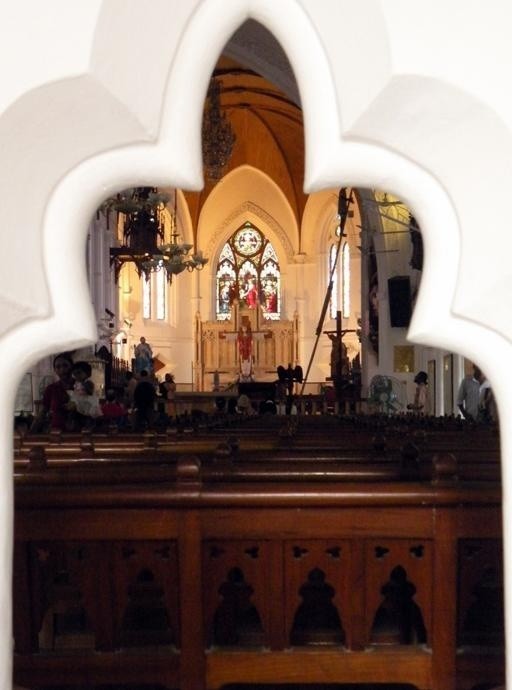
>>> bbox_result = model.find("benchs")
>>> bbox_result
[14,415,503,689]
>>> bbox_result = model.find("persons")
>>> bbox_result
[407,372,428,417]
[134,337,153,373]
[456,364,491,433]
[14,354,176,435]
[325,329,348,377]
[223,323,269,377]
[338,188,349,237]
[230,394,277,417]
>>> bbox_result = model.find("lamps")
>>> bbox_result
[99,187,208,284]
[203,71,237,181]
